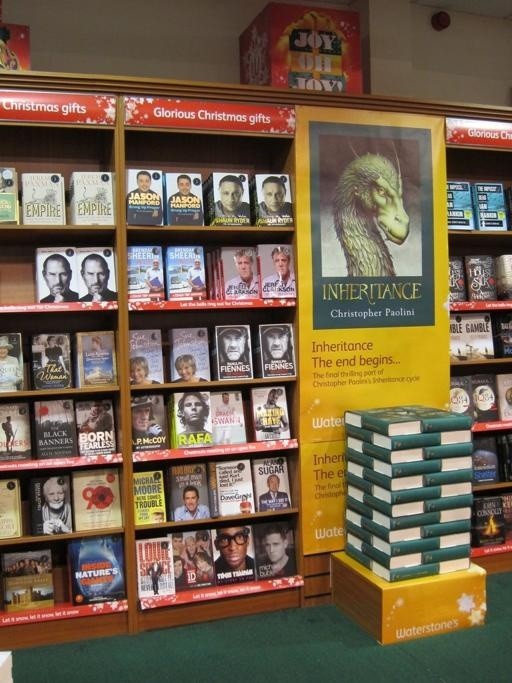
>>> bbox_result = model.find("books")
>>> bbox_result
[126,170,297,302]
[128,323,296,452]
[447,180,512,548]
[132,457,297,598]
[0,468,127,615]
[0,330,118,462]
[0,168,118,304]
[344,404,474,583]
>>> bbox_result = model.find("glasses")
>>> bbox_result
[215,532,248,548]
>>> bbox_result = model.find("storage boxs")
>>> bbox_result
[240,1,364,94]
